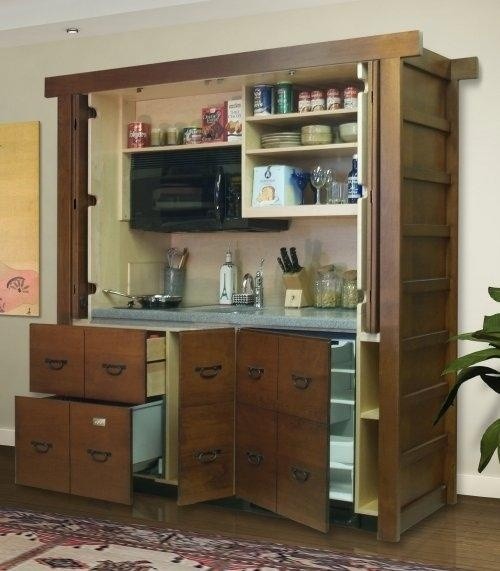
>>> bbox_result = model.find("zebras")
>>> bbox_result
[130,148,290,233]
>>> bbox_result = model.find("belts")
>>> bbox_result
[292,165,335,205]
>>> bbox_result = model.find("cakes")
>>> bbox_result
[183,126,202,145]
[298,91,311,112]
[274,81,295,114]
[127,121,151,148]
[326,88,341,110]
[344,86,358,109]
[311,91,325,111]
[252,84,271,116]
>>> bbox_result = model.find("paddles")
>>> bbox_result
[1,507,463,571]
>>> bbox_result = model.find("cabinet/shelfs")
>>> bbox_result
[11,319,236,521]
[347,155,363,204]
[311,265,363,310]
[163,268,186,296]
[240,82,359,221]
[218,247,237,305]
[239,321,356,534]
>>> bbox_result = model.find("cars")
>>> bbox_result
[336,183,348,203]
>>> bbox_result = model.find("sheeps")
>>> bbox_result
[241,270,264,307]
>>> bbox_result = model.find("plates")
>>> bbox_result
[240,82,359,221]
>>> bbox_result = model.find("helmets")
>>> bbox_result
[261,132,301,149]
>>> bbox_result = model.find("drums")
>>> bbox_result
[275,245,301,274]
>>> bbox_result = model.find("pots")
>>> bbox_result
[102,289,183,310]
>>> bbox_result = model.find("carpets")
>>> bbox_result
[1,507,463,571]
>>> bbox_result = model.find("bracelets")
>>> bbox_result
[300,122,358,144]
